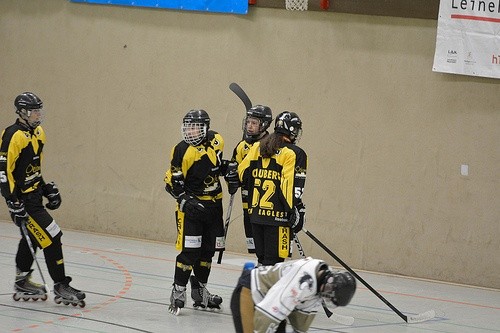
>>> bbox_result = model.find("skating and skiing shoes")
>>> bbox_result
[168,283,186,317]
[13,267,48,302]
[52,276,87,307]
[191,285,224,312]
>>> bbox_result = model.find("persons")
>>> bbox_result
[238,111,308,265]
[0,92,86,308]
[230,258,356,333]
[225,104,274,254]
[163,110,238,317]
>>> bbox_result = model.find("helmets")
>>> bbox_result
[180,109,211,147]
[13,92,46,129]
[241,104,275,139]
[274,111,304,145]
[317,270,357,310]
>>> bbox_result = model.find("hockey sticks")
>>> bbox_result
[216,81,253,265]
[301,226,436,324]
[292,232,355,327]
[21,221,51,302]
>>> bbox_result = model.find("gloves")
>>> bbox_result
[289,203,306,236]
[6,199,29,226]
[41,181,62,211]
[177,195,206,221]
[223,160,240,195]
